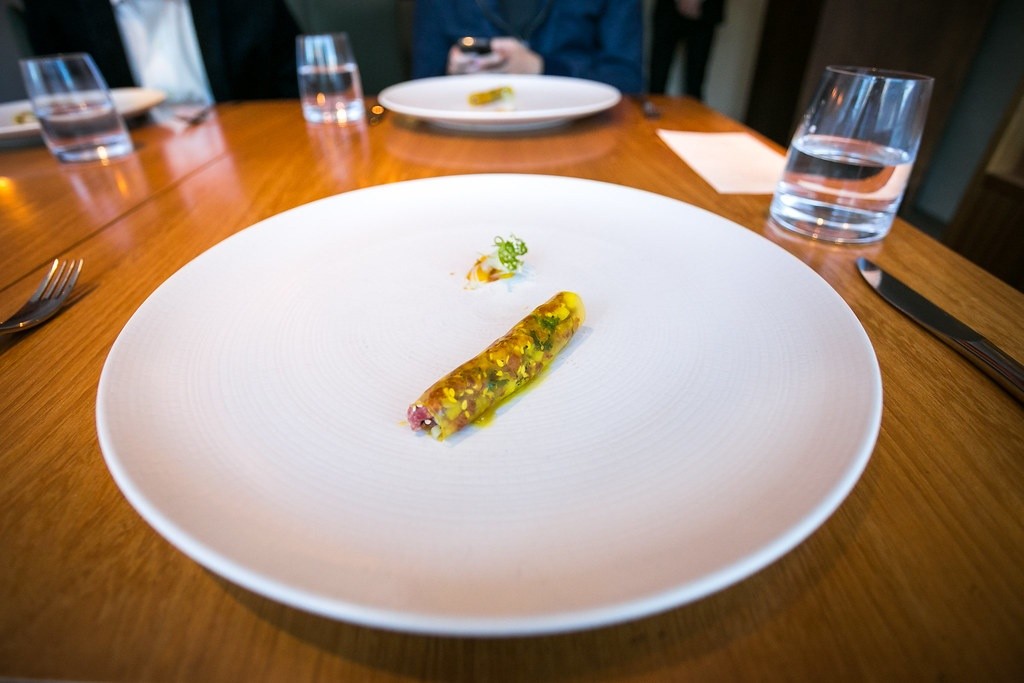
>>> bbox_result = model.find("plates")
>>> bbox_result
[378,74,621,132]
[96,175,882,635]
[0,88,168,150]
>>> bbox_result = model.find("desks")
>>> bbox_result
[0,99,291,290]
[0,94,1024,683]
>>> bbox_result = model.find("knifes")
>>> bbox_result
[856,258,1024,401]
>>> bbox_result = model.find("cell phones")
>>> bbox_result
[459,37,491,55]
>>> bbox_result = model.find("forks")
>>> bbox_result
[0,258,85,336]
[178,107,213,126]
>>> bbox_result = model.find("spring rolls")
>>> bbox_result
[467,87,512,105]
[406,291,586,442]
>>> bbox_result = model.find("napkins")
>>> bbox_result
[655,128,786,195]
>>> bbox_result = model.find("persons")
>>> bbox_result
[649,0,726,104]
[411,0,643,94]
[22,0,306,107]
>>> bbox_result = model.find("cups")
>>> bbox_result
[295,31,365,128]
[20,54,133,168]
[769,66,934,244]
